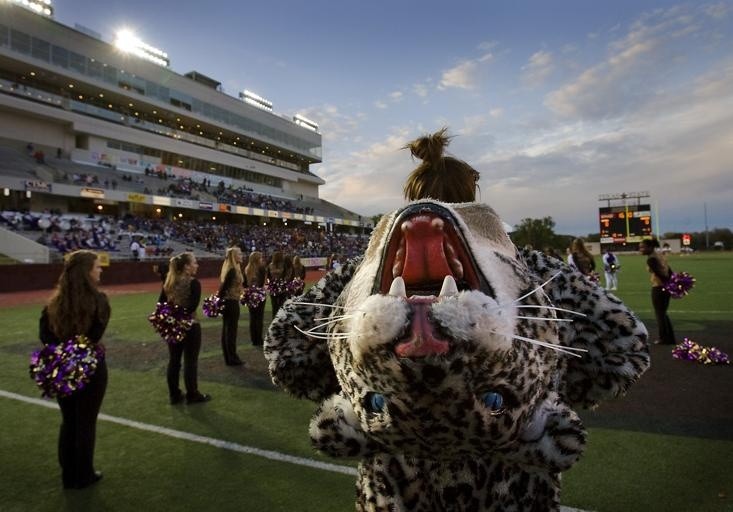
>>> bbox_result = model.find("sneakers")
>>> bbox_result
[200,392,211,401]
[94,470,102,480]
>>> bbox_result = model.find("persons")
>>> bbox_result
[267,249,289,320]
[243,249,267,347]
[36,247,114,491]
[292,255,306,298]
[1,141,371,271]
[541,244,565,264]
[152,247,211,407]
[215,245,245,366]
[601,245,621,292]
[637,236,677,347]
[568,237,597,283]
[283,254,294,299]
[259,124,655,511]
